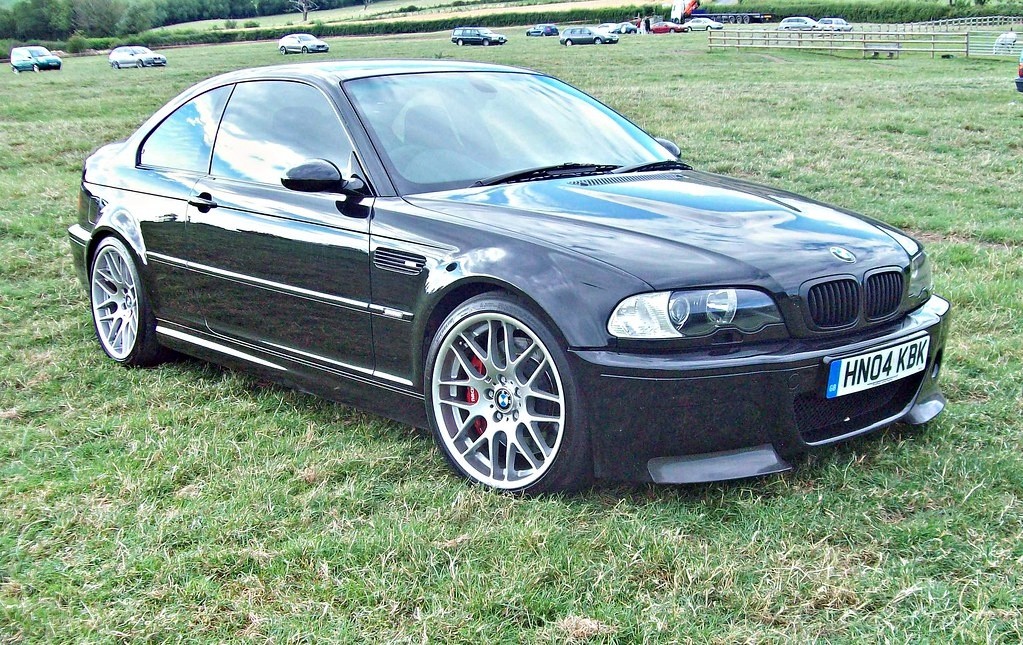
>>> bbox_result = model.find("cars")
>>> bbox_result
[109,47,167,69]
[816,18,854,32]
[277,34,327,55]
[560,27,620,47]
[597,22,638,34]
[450,26,508,46]
[652,20,687,31]
[9,47,61,71]
[780,17,820,31]
[685,17,723,30]
[525,24,561,36]
[63,59,953,497]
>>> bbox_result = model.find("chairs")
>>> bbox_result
[404,104,463,156]
[268,106,350,167]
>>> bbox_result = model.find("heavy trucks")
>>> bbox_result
[671,1,774,23]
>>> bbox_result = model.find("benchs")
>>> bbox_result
[863,45,902,59]
